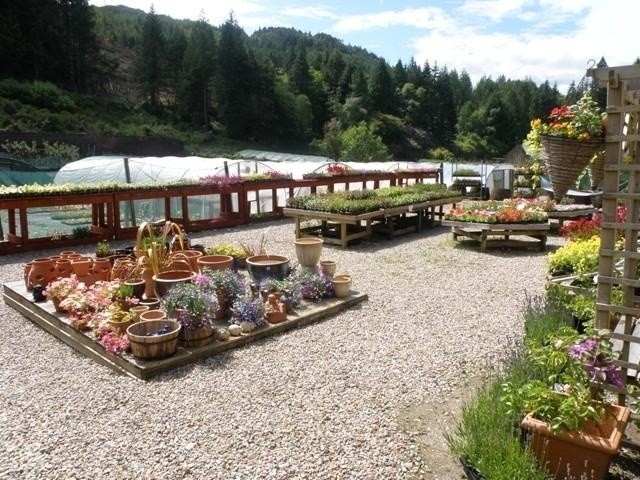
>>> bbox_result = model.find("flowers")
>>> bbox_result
[522,96,608,162]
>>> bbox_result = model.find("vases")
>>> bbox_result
[21,218,356,362]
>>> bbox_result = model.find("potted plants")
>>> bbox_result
[445,198,638,480]
[511,164,546,199]
[1,160,483,245]
